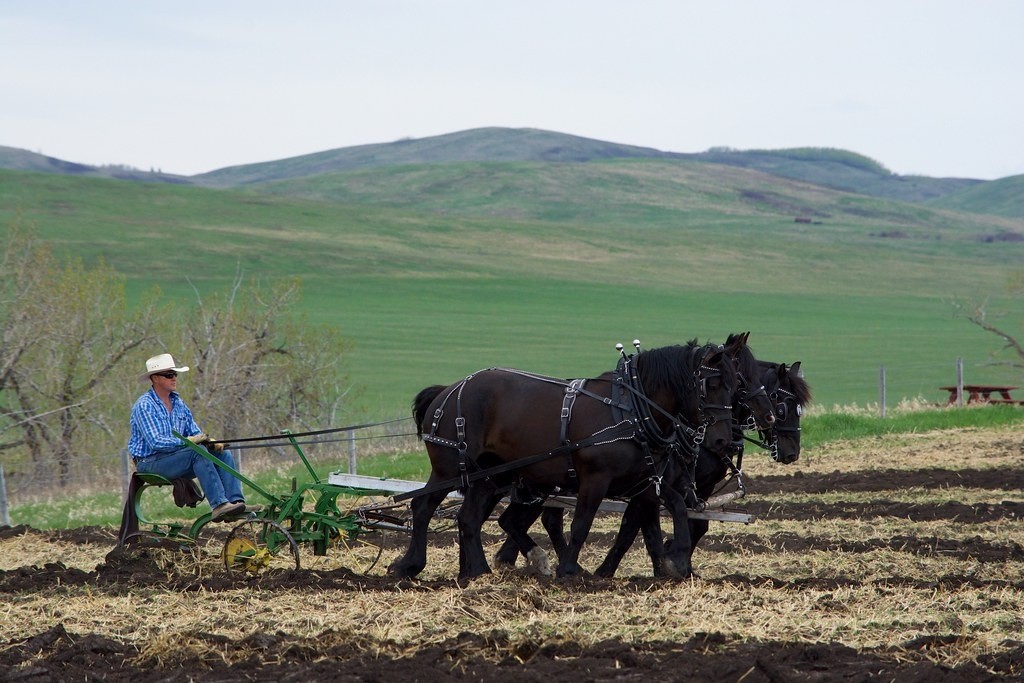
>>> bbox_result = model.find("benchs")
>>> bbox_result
[990,399,1024,403]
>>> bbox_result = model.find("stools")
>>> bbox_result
[134,472,173,488]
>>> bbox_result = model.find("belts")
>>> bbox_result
[133,456,142,465]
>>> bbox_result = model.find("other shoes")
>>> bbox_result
[211,502,246,523]
[223,501,263,523]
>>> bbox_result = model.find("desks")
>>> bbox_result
[938,385,1020,404]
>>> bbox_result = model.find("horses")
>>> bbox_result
[386,330,814,589]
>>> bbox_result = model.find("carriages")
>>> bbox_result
[103,331,813,591]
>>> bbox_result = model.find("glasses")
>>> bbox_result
[156,372,177,378]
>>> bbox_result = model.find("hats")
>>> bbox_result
[136,354,190,378]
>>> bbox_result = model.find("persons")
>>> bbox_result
[128,353,264,523]
